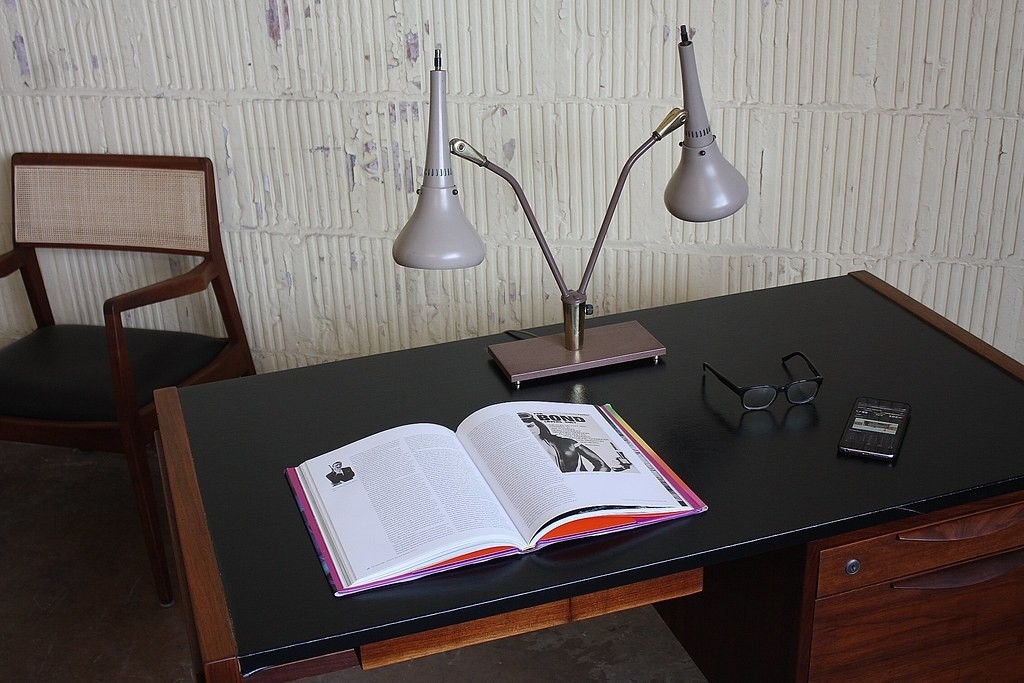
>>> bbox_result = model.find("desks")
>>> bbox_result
[150,270,1024,683]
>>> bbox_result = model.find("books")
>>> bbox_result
[285,401,708,596]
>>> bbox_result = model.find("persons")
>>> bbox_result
[325,462,354,487]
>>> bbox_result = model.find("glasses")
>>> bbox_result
[702,350,825,410]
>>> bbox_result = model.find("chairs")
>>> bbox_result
[0,152,257,606]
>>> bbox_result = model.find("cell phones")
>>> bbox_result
[838,397,912,461]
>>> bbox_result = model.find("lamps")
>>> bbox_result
[392,24,749,390]
